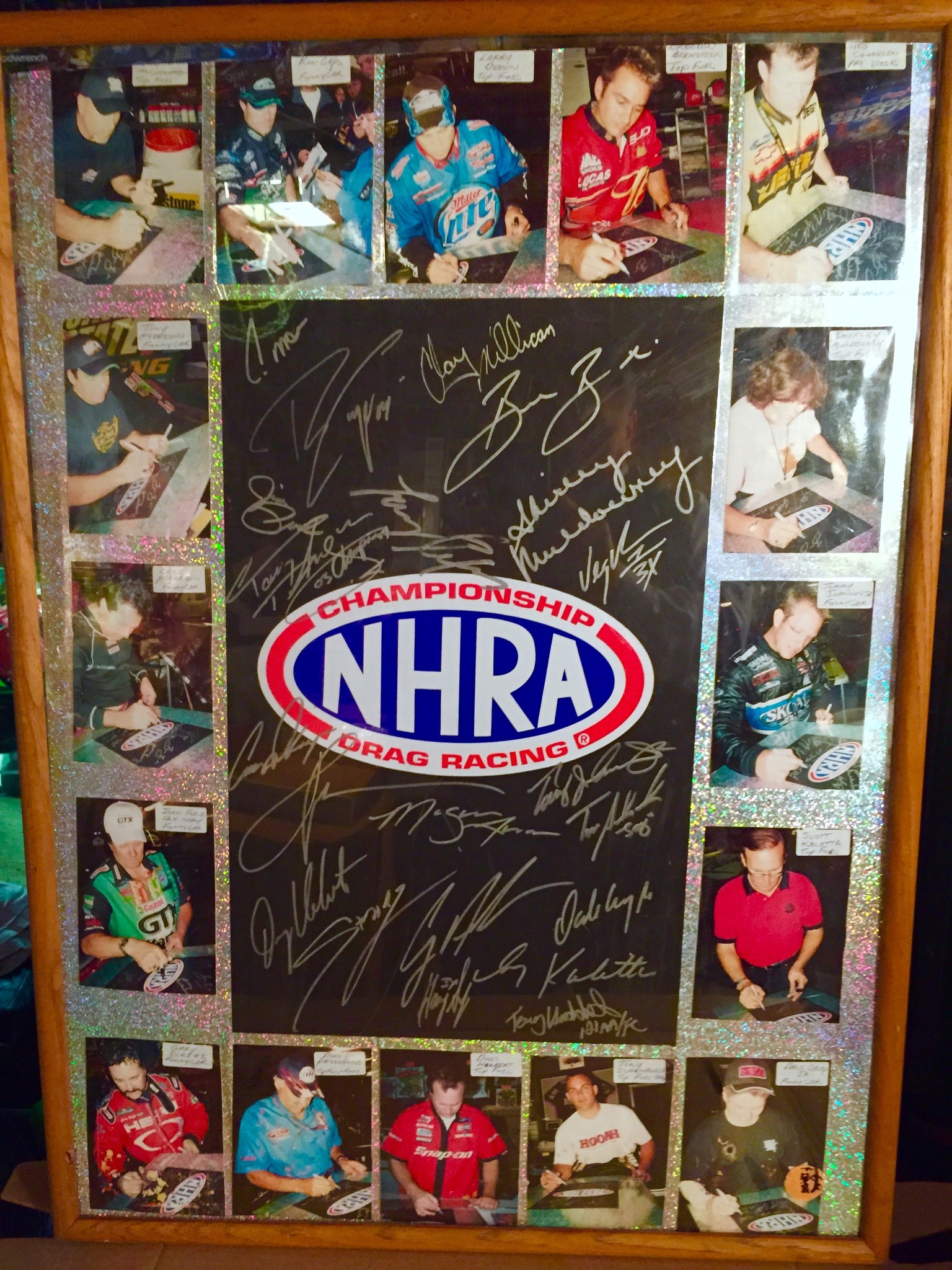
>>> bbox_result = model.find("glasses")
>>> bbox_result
[745,863,783,879]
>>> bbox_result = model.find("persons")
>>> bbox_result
[54,45,849,1233]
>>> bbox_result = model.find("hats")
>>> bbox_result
[79,68,130,114]
[63,335,120,377]
[238,74,284,110]
[402,74,451,128]
[277,1055,324,1099]
[722,1058,774,1094]
[103,801,147,843]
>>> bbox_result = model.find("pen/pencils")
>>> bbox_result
[137,700,162,723]
[592,232,630,275]
[127,442,160,464]
[775,511,805,539]
[433,253,467,281]
[772,748,808,769]
[546,1169,569,1186]
[715,1188,743,1215]
[818,704,833,722]
[752,985,765,1012]
[160,964,164,977]
[313,1174,341,1189]
[120,207,153,232]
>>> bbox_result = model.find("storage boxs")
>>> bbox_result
[140,165,204,209]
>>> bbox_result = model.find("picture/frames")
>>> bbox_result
[1,0,952,1269]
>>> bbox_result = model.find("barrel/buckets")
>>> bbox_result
[141,128,200,171]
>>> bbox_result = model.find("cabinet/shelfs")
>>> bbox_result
[652,107,728,210]
